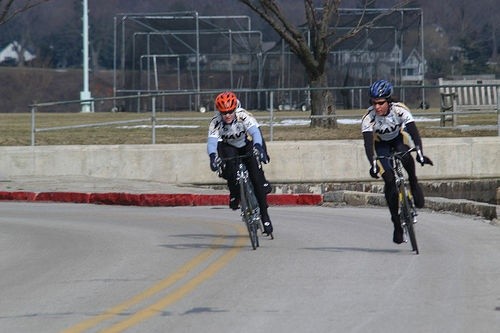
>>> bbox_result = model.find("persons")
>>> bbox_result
[208,92,273,235]
[361,80,434,244]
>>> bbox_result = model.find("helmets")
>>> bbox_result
[215,92,237,111]
[368,80,393,98]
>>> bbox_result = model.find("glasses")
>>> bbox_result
[372,100,387,105]
[221,110,235,115]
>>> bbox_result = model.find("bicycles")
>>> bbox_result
[216,147,268,250]
[373,145,424,255]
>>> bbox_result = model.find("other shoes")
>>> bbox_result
[393,224,405,244]
[229,196,242,208]
[261,217,273,234]
[263,182,272,193]
[409,184,424,207]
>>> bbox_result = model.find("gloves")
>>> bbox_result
[254,143,267,161]
[415,155,433,165]
[370,165,381,179]
[210,155,219,171]
